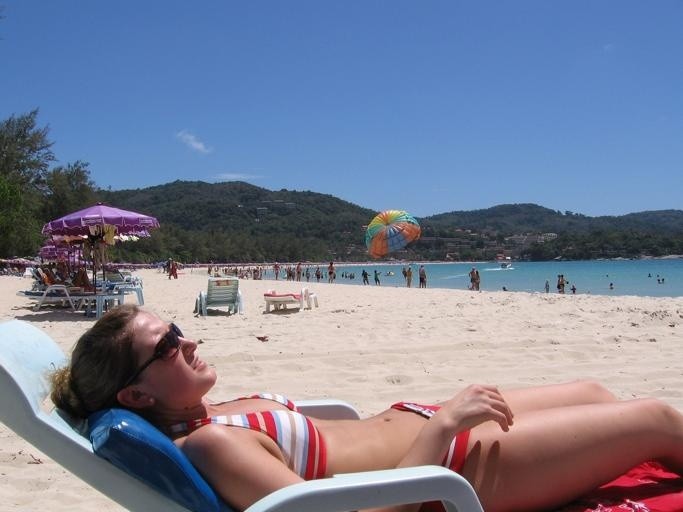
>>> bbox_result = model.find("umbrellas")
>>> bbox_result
[40,202,160,313]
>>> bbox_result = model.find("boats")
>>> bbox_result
[499,263,512,269]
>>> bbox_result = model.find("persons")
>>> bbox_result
[341,268,382,286]
[145,257,185,280]
[544,274,576,294]
[647,273,665,284]
[502,263,511,269]
[468,267,480,291]
[4,257,136,294]
[402,264,427,289]
[274,262,336,284]
[207,264,264,280]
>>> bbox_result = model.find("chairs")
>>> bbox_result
[18,264,145,317]
[264,288,319,312]
[196,277,244,316]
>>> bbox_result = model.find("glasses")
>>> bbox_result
[127,323,184,388]
